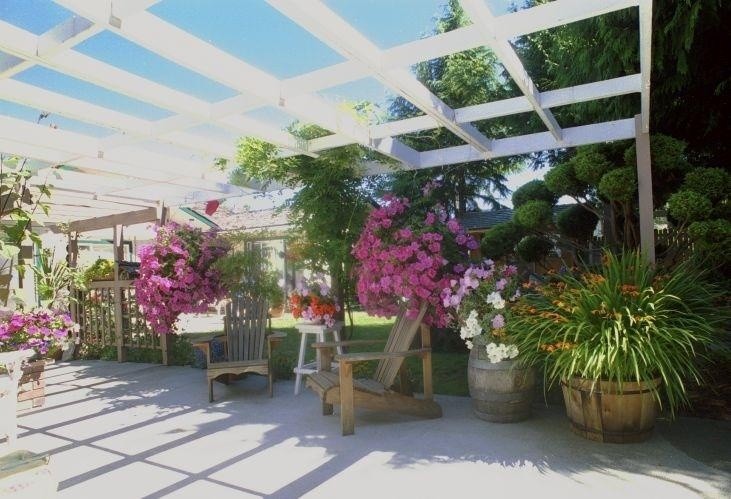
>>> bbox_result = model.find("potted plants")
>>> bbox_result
[504,240,730,443]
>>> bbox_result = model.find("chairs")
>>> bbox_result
[192,296,287,403]
[307,297,442,437]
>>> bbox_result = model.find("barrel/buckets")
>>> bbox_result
[560,373,660,443]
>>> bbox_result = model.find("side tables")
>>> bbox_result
[293,324,344,395]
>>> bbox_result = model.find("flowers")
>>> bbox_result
[1,309,82,355]
[134,220,233,335]
[284,190,479,329]
[444,257,530,365]
